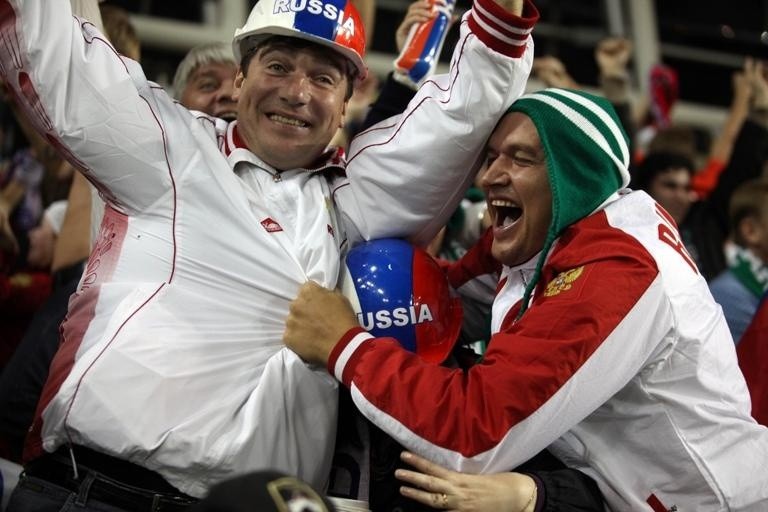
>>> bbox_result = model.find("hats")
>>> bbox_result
[512,87,633,233]
[199,470,336,511]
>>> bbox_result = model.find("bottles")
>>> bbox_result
[393,0,458,86]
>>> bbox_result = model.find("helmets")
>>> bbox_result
[336,238,464,368]
[230,0,367,84]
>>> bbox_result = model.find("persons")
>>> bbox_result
[1,0,767,512]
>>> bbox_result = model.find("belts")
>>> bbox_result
[25,456,191,512]
